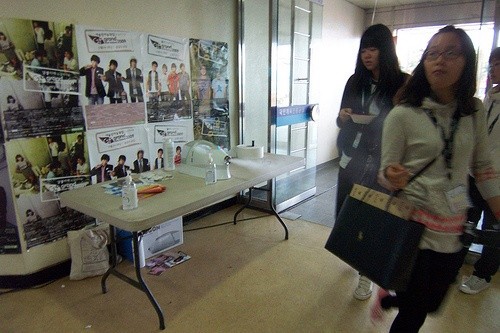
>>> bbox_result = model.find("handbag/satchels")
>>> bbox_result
[325,168,429,292]
[338,131,370,175]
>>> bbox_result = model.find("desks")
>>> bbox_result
[60,152,304,328]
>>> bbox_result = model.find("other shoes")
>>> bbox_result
[355,275,373,299]
[370,286,389,321]
[459,275,490,294]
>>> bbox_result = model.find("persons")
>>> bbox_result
[7,95,19,110]
[63,50,77,78]
[117,58,144,102]
[57,26,70,41]
[450,48,500,293]
[194,64,226,140]
[79,55,106,105]
[42,84,52,107]
[33,21,46,57]
[0,32,20,70]
[25,209,50,238]
[337,24,500,332]
[48,40,59,68]
[15,135,88,189]
[44,30,55,51]
[90,146,181,184]
[146,61,191,121]
[102,60,124,103]
[31,53,42,73]
[51,82,79,106]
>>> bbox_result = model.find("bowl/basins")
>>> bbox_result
[350,114,376,125]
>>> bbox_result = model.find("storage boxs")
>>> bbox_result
[116,216,184,262]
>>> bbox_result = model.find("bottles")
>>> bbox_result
[163,139,176,171]
[122,170,138,210]
[205,152,217,186]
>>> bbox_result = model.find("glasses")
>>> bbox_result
[424,48,467,63]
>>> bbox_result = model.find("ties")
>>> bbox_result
[139,160,143,167]
